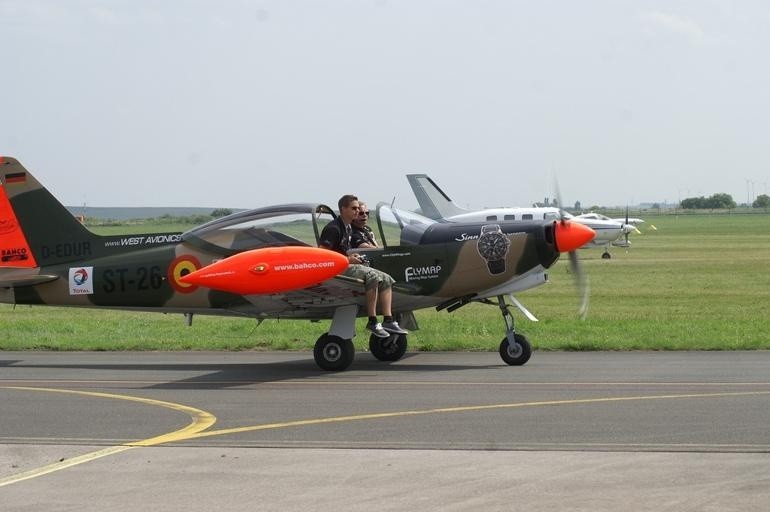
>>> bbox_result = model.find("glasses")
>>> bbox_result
[358,212,369,215]
[351,207,361,210]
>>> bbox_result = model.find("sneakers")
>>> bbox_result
[365,321,408,337]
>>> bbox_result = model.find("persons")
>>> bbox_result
[318,194,408,338]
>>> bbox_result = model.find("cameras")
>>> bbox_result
[356,255,370,262]
